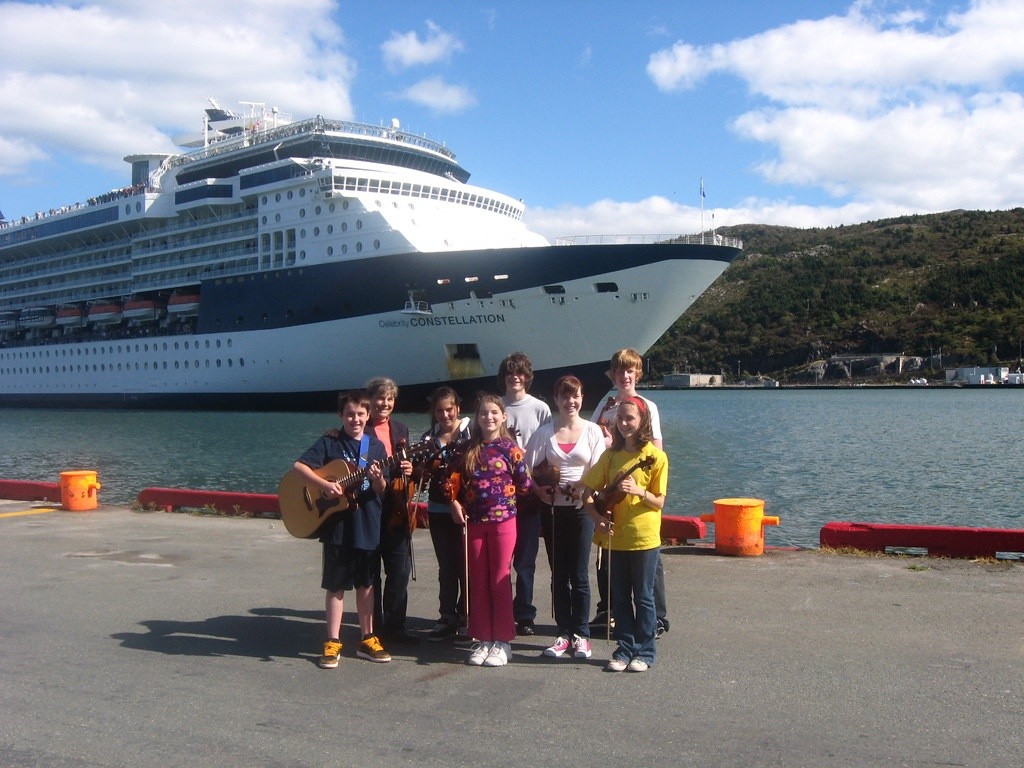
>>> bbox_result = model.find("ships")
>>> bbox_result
[0,95,747,414]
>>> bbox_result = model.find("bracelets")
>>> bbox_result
[641,488,648,503]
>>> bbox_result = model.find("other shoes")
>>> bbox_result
[428,618,458,641]
[383,630,418,645]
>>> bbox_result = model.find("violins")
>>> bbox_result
[593,454,658,518]
[430,417,471,504]
[386,437,417,538]
[596,396,616,433]
[531,458,579,502]
[410,441,457,481]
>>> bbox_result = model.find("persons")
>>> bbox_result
[443,394,532,667]
[405,280,498,310]
[338,377,424,647]
[588,348,669,640]
[490,351,554,636]
[581,395,670,672]
[402,386,482,645]
[0,172,186,232]
[293,391,391,668]
[312,158,334,171]
[252,122,264,135]
[523,375,608,660]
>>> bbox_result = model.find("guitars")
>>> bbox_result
[279,435,434,538]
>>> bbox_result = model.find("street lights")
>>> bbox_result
[737,360,741,379]
[647,359,650,374]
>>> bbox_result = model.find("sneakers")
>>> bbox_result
[544,636,570,657]
[321,638,342,669]
[357,634,391,662]
[518,622,536,635]
[470,641,492,665]
[484,641,512,667]
[608,659,628,671]
[628,660,648,672]
[571,634,592,657]
